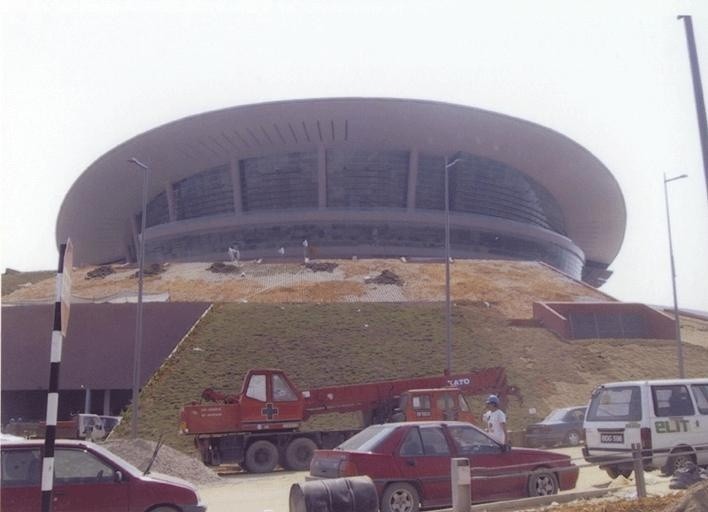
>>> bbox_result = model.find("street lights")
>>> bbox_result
[442,153,462,373]
[302,239,309,259]
[279,247,286,258]
[663,171,690,379]
[80,384,85,413]
[124,156,148,439]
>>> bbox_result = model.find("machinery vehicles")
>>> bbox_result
[180,365,525,474]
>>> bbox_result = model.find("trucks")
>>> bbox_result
[38,414,105,439]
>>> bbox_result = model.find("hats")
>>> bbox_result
[486,395,499,403]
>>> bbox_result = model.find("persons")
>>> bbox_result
[479,394,509,448]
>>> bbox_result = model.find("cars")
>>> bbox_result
[305,421,580,512]
[1,440,206,510]
[524,405,590,448]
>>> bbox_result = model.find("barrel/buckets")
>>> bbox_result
[289,475,379,512]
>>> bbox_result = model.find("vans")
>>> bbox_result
[582,378,708,479]
